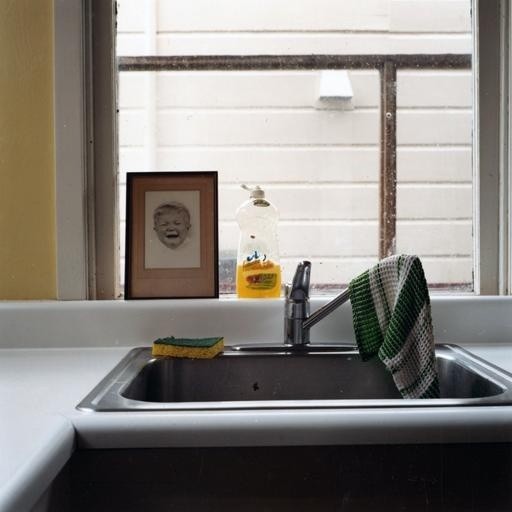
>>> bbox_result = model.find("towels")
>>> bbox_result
[346,254,441,398]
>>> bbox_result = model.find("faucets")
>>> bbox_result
[281,260,351,349]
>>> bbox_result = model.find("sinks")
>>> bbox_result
[74,342,512,412]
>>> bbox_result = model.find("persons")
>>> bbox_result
[146,200,200,269]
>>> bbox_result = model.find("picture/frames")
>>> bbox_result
[124,170,220,301]
